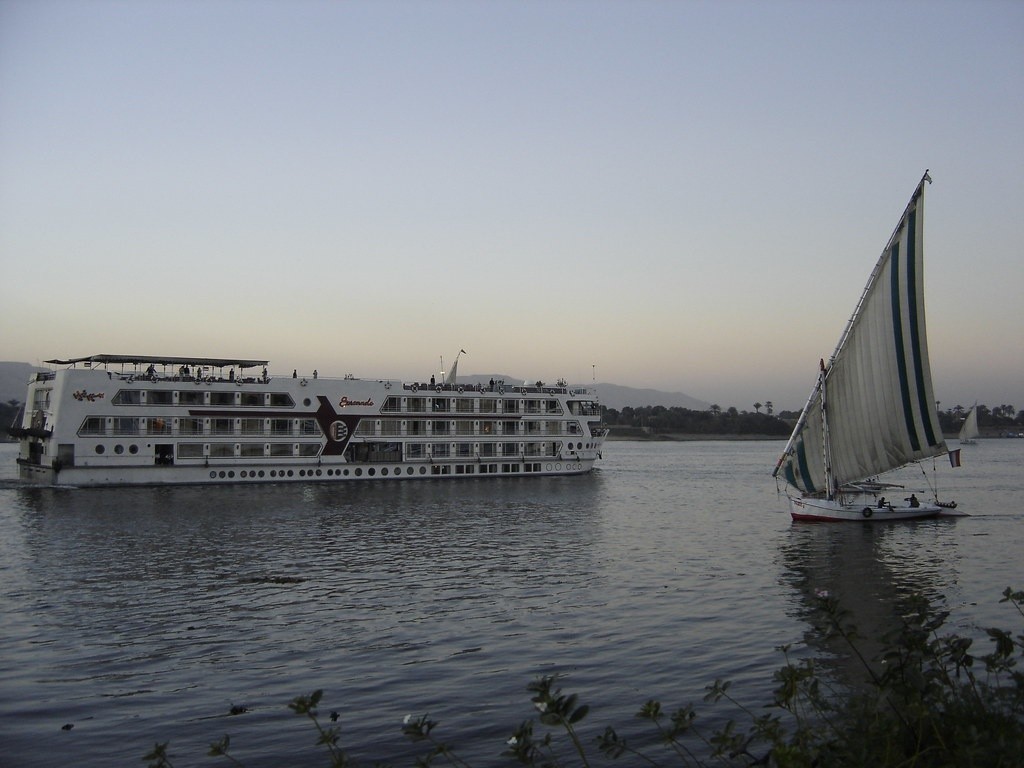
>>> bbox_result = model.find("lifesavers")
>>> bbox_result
[521,388,528,395]
[862,506,873,518]
[435,385,443,393]
[151,375,159,384]
[549,389,556,396]
[499,388,505,395]
[125,374,135,384]
[384,383,392,389]
[236,378,244,386]
[457,386,464,395]
[205,377,213,385]
[411,385,418,393]
[480,387,486,394]
[194,377,202,385]
[299,378,309,387]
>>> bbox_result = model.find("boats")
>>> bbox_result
[4,353,614,491]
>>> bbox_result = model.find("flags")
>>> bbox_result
[949,450,962,468]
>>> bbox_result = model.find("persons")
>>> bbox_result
[230,368,234,382]
[179,365,190,381]
[262,368,267,383]
[145,364,156,380]
[313,370,318,379]
[197,367,202,380]
[878,497,886,508]
[430,374,435,389]
[292,369,298,378]
[489,377,495,392]
[590,402,596,415]
[535,381,543,393]
[905,494,920,508]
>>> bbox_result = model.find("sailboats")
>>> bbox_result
[956,402,981,446]
[766,165,964,525]
